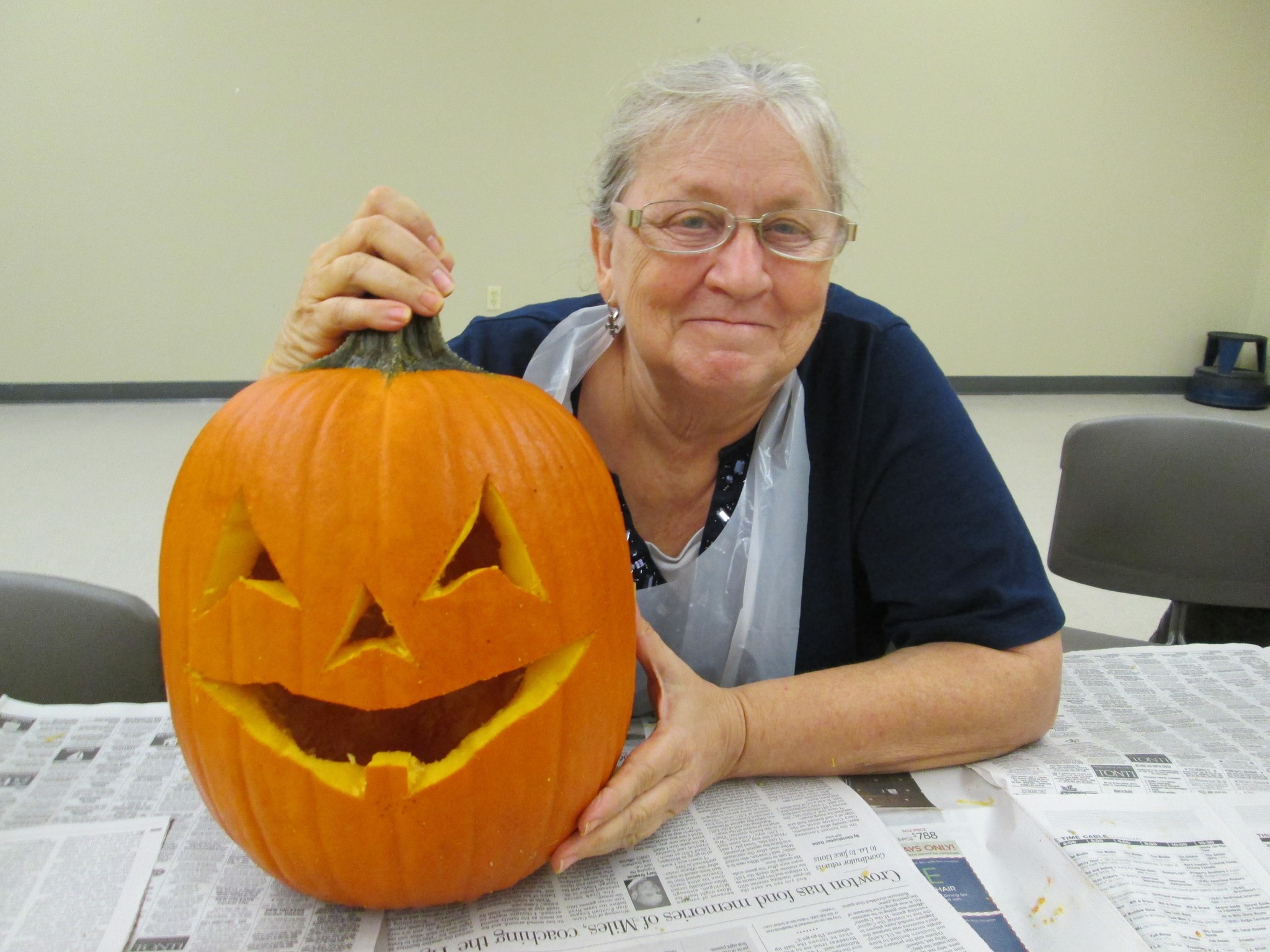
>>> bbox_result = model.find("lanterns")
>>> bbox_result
[150,264,641,914]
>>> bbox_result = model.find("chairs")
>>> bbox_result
[1,570,167,706]
[1043,414,1270,650]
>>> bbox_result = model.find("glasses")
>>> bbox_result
[608,200,857,261]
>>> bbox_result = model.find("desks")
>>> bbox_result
[0,645,1270,952]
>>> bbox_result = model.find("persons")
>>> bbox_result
[250,56,1071,873]
